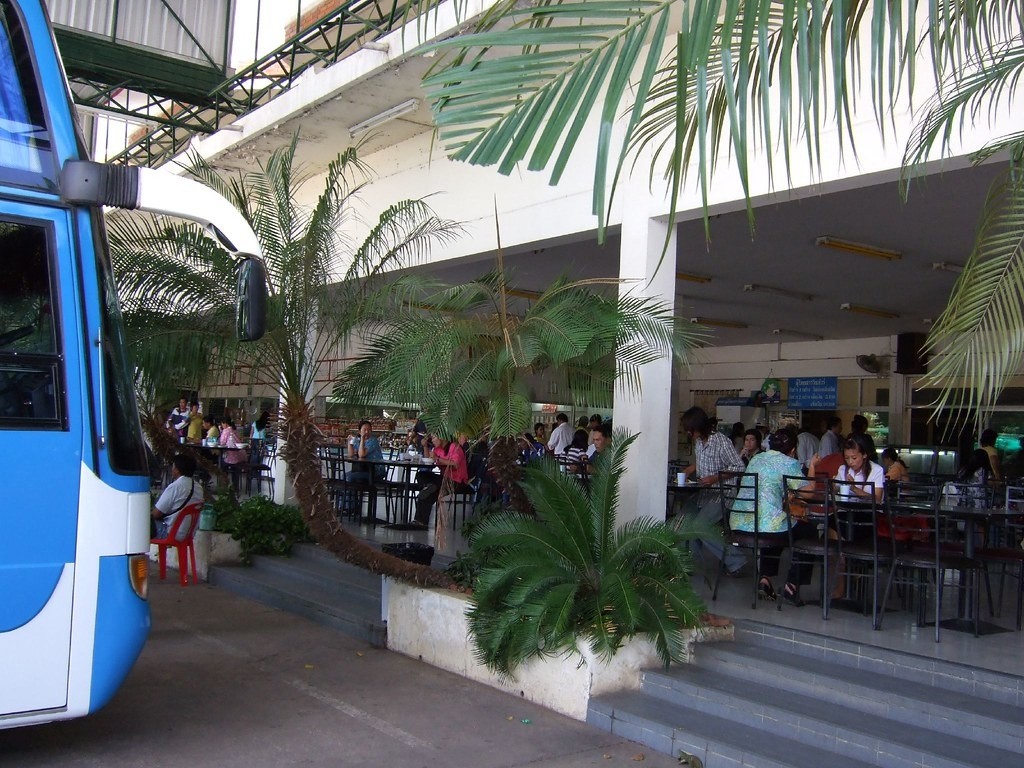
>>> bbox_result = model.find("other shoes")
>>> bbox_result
[333,508,354,516]
[408,520,428,530]
[721,562,749,577]
[839,588,865,611]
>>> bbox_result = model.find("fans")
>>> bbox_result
[856,353,887,380]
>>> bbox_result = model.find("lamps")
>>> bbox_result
[815,236,902,262]
[503,286,543,300]
[401,301,463,313]
[689,317,749,329]
[841,302,900,320]
[922,318,942,325]
[745,284,813,303]
[936,264,966,274]
[676,272,712,284]
[773,328,823,342]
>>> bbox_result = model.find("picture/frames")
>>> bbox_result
[601,369,614,392]
[470,334,515,382]
[569,363,591,390]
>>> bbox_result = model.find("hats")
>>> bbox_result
[756,422,765,426]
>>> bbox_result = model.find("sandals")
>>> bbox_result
[783,581,805,607]
[758,577,776,600]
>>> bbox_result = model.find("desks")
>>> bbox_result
[372,460,455,531]
[338,456,396,526]
[795,495,1024,628]
[196,446,248,494]
[668,484,733,575]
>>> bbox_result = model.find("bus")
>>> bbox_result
[2,0,268,729]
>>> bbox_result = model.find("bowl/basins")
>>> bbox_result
[207,442,218,447]
[235,443,249,448]
[422,458,436,463]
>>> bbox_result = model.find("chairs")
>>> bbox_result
[150,501,206,586]
[241,436,592,534]
[711,470,1024,647]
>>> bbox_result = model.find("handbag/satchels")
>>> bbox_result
[416,472,441,493]
[151,518,157,538]
[939,481,959,507]
[782,488,809,523]
[877,507,930,544]
[351,454,375,472]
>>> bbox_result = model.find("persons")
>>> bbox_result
[149,454,205,541]
[166,392,1000,607]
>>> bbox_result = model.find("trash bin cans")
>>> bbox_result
[380,541,435,623]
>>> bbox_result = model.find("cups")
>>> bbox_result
[181,437,185,443]
[399,453,405,460]
[840,485,850,501]
[202,439,206,446]
[677,473,685,486]
[418,455,423,463]
